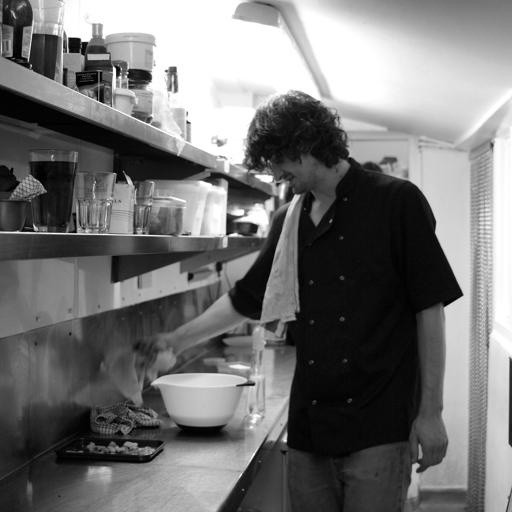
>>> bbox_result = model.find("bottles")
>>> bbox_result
[1,1,192,144]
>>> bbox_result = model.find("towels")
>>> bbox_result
[259,190,303,338]
[72,346,178,411]
[89,399,161,436]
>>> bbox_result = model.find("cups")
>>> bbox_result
[132,181,155,234]
[74,172,118,233]
[28,150,80,232]
[217,335,267,433]
[249,375,265,419]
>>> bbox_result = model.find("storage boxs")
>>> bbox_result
[145,180,227,236]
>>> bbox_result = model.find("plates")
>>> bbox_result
[222,336,266,347]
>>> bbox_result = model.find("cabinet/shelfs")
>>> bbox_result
[179,143,278,276]
[0,50,218,285]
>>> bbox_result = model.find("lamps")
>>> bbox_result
[232,2,297,55]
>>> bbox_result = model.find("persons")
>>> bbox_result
[135,87,463,511]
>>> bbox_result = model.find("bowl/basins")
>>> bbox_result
[149,205,186,235]
[1,199,32,232]
[151,372,255,433]
